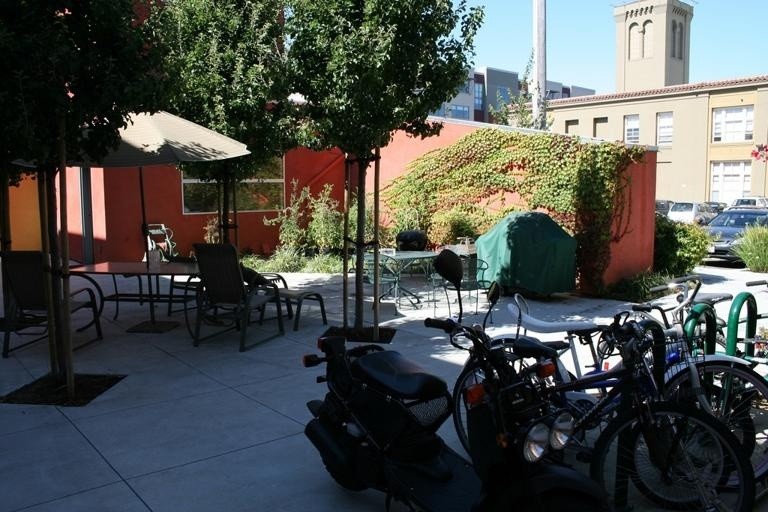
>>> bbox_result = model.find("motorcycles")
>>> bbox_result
[304,249,609,512]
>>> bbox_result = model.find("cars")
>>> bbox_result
[655,197,768,262]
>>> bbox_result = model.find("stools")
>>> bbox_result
[257,270,327,331]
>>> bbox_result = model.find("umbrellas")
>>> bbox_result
[8,108,252,269]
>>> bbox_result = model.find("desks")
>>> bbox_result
[369,249,439,319]
[65,261,243,344]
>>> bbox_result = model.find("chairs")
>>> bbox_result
[146,221,284,353]
[0,246,107,360]
[431,258,497,325]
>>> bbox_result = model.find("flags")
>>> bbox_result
[750,142,767,166]
